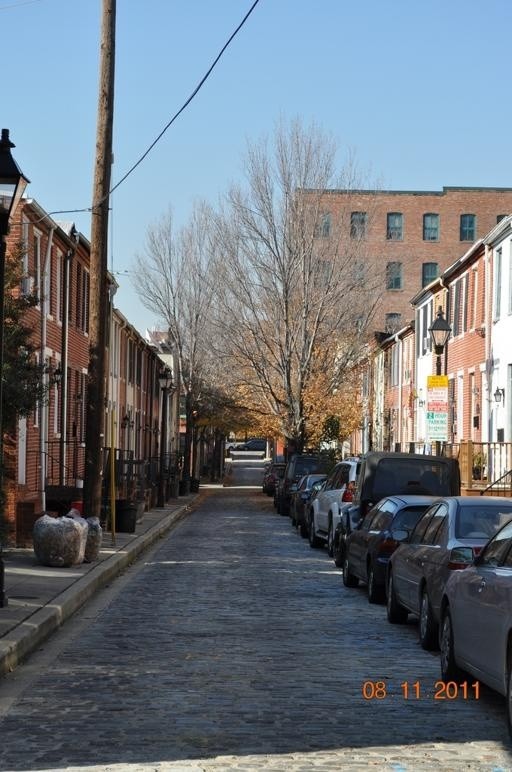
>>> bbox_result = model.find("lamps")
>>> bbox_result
[494,387,505,407]
[143,423,150,432]
[50,361,63,387]
[419,399,425,407]
[121,415,130,428]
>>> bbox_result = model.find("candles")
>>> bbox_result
[473,450,487,480]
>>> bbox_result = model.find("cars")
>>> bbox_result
[436,517,512,735]
[237,440,265,451]
[384,495,511,650]
[224,441,236,451]
[264,451,463,567]
[339,494,446,606]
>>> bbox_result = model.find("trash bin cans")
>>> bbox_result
[179,480,190,496]
[115,499,138,533]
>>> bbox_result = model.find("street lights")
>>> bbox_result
[156,365,172,507]
[428,304,452,456]
